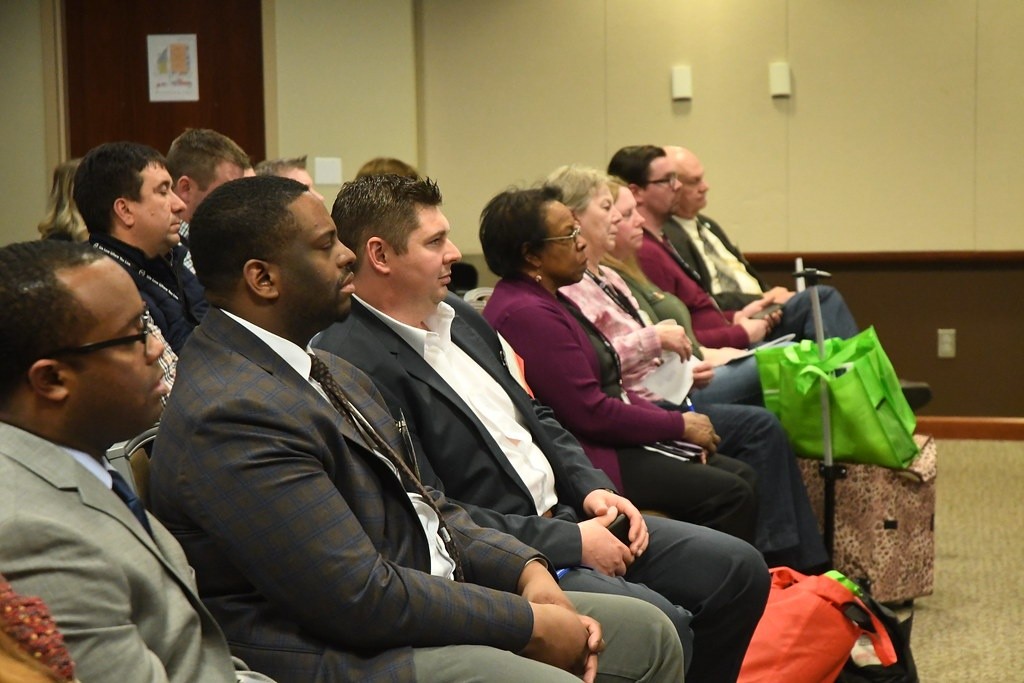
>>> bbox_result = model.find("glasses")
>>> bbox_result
[647,170,678,191]
[542,225,582,251]
[56,303,151,365]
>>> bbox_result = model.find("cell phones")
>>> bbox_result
[607,513,634,549]
[749,303,783,319]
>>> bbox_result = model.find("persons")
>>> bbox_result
[0,122,925,683]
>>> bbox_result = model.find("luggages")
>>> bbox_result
[788,267,936,605]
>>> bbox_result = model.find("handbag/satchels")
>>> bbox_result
[755,328,923,475]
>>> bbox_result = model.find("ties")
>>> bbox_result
[307,353,462,584]
[695,221,741,294]
[104,466,155,539]
